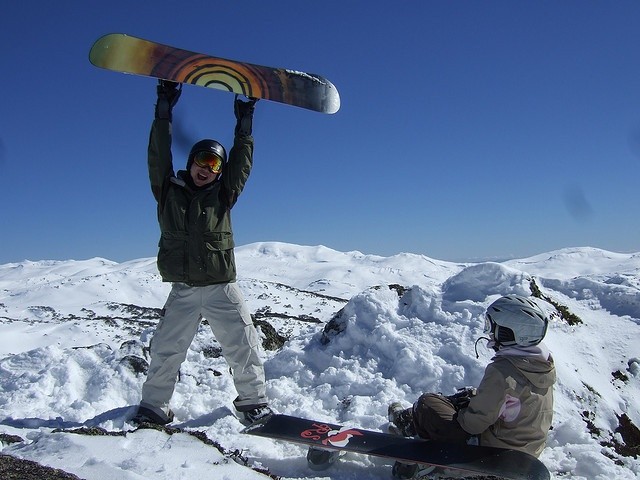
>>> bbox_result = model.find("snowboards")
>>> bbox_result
[240,413,550,480]
[89,33,341,115]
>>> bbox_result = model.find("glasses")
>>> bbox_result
[482,312,497,335]
[193,151,224,173]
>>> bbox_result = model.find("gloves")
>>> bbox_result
[455,388,472,410]
[234,99,255,137]
[155,84,181,123]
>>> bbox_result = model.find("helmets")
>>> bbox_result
[487,296,548,350]
[187,140,227,170]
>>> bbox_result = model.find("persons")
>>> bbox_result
[385,296,558,480]
[119,78,275,430]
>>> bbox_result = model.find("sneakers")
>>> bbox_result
[388,402,417,437]
[126,406,173,427]
[244,406,275,423]
